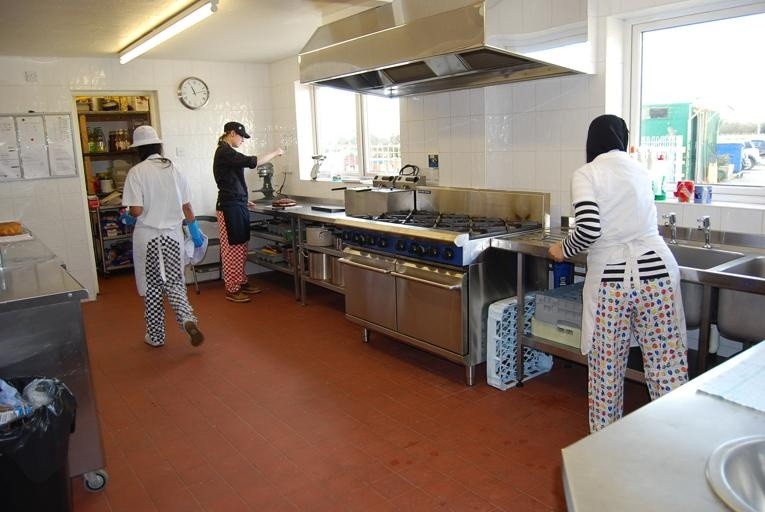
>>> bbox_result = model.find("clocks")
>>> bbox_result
[177,76,209,110]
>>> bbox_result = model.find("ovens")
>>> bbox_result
[337,251,468,367]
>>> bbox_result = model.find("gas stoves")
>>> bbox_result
[336,210,540,267]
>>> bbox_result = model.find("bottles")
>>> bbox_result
[110,129,130,151]
[95,127,106,152]
[87,127,95,152]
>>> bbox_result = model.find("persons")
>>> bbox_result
[213,121,284,304]
[549,115,690,434]
[117,125,206,347]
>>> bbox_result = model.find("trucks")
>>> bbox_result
[640,103,718,189]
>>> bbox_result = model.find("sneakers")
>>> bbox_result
[144,333,164,346]
[225,283,263,301]
[183,319,203,346]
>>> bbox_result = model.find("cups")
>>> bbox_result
[677,181,694,202]
[694,186,712,203]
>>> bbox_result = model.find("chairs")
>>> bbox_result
[183,215,222,294]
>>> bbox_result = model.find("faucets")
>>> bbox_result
[696,214,712,249]
[661,212,678,244]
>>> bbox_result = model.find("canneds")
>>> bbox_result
[694,185,714,204]
[675,181,694,201]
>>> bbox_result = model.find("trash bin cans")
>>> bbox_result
[1,376,76,511]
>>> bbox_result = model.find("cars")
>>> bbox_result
[719,133,765,170]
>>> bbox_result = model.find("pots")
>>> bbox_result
[300,251,332,280]
[305,224,334,247]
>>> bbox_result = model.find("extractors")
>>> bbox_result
[297,0,596,97]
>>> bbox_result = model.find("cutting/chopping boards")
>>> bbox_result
[263,205,302,211]
[311,205,345,213]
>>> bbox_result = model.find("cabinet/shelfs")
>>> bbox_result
[247,199,346,306]
[77,95,148,279]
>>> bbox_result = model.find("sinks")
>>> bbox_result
[665,244,746,270]
[715,253,765,273]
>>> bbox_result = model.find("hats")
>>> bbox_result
[128,126,164,148]
[224,122,251,138]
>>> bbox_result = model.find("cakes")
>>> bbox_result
[272,198,298,208]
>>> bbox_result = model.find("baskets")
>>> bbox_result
[487,290,553,392]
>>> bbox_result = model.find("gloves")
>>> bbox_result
[119,211,136,225]
[187,220,204,247]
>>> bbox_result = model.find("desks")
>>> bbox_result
[0,223,109,492]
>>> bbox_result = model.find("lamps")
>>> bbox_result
[118,0,218,64]
